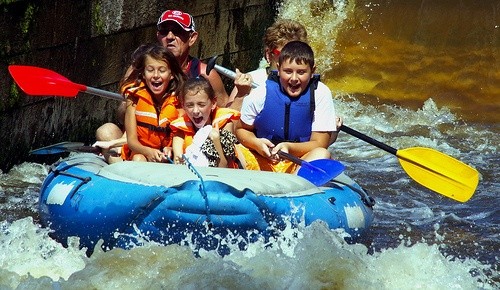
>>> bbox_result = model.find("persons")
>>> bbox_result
[95,3,307,176]
[234,40,338,175]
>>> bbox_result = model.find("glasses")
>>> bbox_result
[157,26,185,37]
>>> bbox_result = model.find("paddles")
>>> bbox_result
[8,64,124,100]
[268,145,346,187]
[29,140,103,154]
[206,54,480,203]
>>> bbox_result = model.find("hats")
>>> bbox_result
[157,10,195,32]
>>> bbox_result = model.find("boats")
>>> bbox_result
[38,153,376,260]
